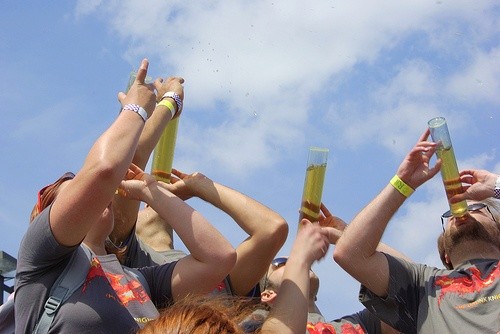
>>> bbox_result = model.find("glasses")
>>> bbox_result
[263,258,292,289]
[38,185,48,213]
[441,203,496,232]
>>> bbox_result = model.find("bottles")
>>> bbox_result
[120,72,152,111]
[297,148,328,233]
[151,114,180,183]
[429,117,468,220]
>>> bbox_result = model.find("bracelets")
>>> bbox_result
[390,175,414,198]
[160,91,182,113]
[494,175,500,199]
[157,101,175,119]
[120,104,148,123]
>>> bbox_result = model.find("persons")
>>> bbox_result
[0,58,416,334]
[332,128,500,334]
